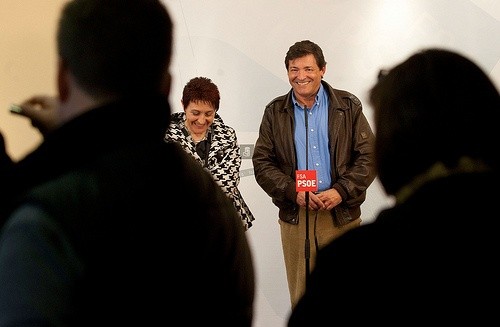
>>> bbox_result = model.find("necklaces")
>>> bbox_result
[183,121,211,143]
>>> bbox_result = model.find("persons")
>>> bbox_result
[165,77,256,232]
[287,48,499,327]
[0,0,255,326]
[252,39,377,312]
